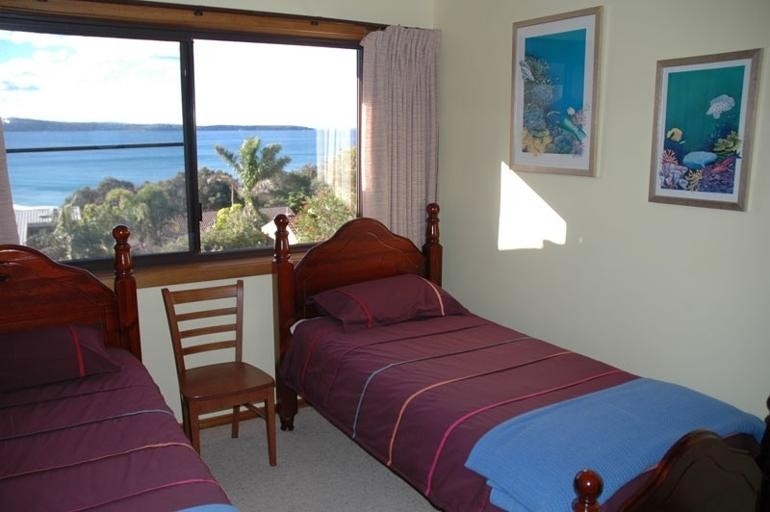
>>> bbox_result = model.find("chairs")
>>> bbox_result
[161,280,277,467]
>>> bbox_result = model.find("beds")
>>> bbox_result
[0,224,237,512]
[272,202,770,512]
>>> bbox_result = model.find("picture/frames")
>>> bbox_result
[510,6,606,176]
[648,48,763,212]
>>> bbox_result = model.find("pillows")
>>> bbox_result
[312,274,470,334]
[0,321,121,394]
